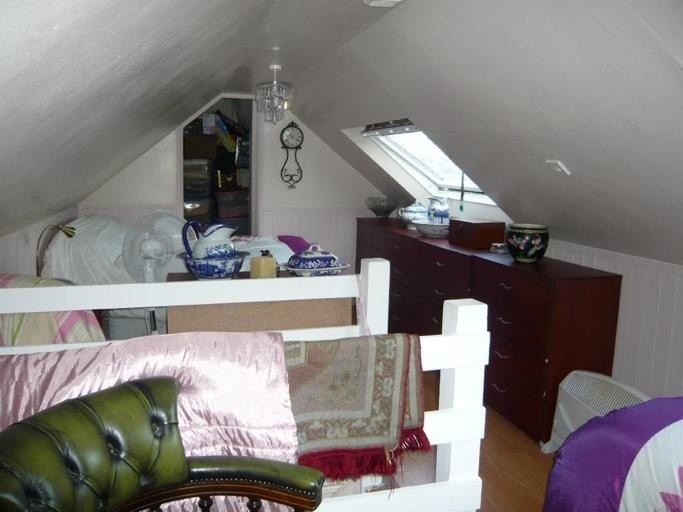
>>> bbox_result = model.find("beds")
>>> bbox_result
[29,214,310,286]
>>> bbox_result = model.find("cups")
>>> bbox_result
[250,256,276,278]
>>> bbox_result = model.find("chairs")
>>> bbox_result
[0,373,327,512]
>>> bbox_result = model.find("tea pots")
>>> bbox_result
[397,199,436,231]
[427,196,450,225]
[181,220,238,258]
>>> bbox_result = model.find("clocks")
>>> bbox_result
[278,119,305,189]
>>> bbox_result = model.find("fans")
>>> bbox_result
[119,209,197,281]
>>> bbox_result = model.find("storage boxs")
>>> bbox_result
[446,216,505,252]
[182,131,249,237]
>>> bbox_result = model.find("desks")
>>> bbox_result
[160,266,353,340]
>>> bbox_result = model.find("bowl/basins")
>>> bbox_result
[175,250,251,280]
[505,222,549,264]
[410,219,449,238]
[489,242,508,255]
[279,243,350,277]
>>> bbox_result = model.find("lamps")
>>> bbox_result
[33,220,78,280]
[250,55,297,123]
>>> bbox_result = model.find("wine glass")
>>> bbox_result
[365,197,398,224]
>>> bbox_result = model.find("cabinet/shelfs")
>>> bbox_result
[470,254,623,442]
[352,215,381,273]
[410,240,471,337]
[381,228,410,335]
